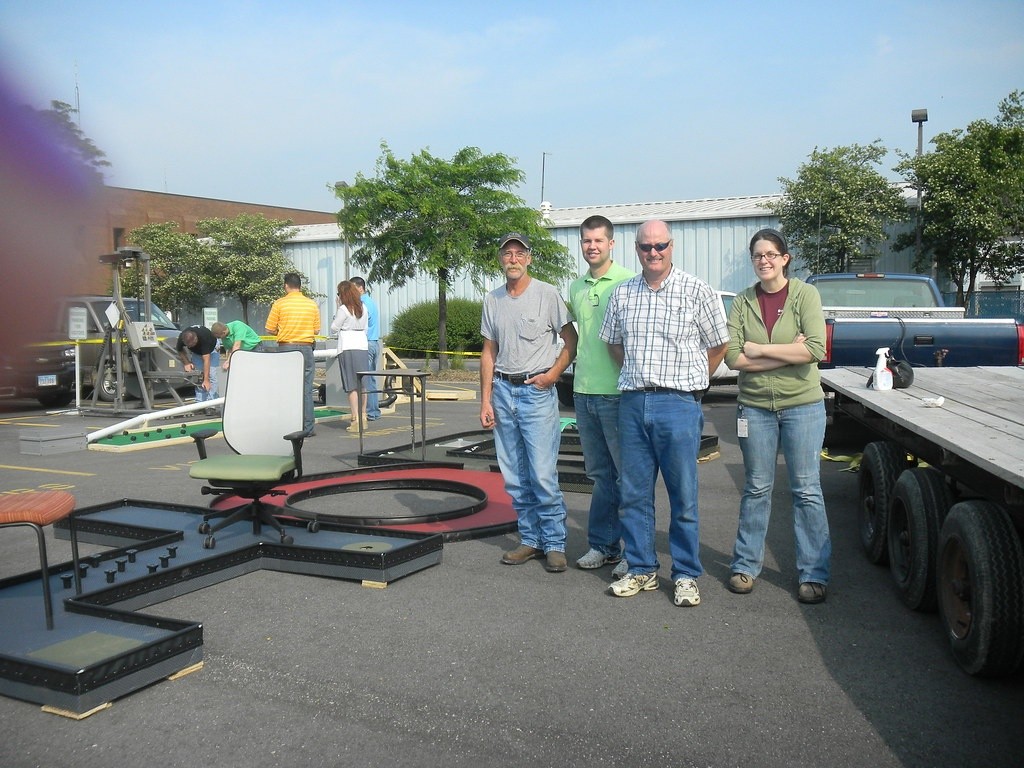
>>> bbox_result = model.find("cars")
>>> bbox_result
[556,291,747,393]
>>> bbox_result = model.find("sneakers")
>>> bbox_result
[798,579,827,603]
[607,571,661,596]
[727,573,754,593]
[546,550,567,571]
[612,559,629,578]
[576,549,622,568]
[500,545,545,564]
[674,576,701,607]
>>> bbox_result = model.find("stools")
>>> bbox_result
[0,491,80,631]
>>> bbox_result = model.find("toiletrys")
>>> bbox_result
[874,345,894,390]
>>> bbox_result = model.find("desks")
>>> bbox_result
[357,369,431,459]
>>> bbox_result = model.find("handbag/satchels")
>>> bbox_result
[866,357,915,389]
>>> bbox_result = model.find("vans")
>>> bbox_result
[19,297,202,411]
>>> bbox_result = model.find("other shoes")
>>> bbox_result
[376,416,380,420]
[304,430,316,437]
[206,407,216,415]
[366,414,375,420]
[194,409,206,414]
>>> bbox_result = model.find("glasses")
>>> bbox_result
[637,239,673,252]
[751,252,784,262]
[499,249,529,260]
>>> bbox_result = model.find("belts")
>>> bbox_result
[278,342,314,347]
[494,369,549,387]
[642,385,671,393]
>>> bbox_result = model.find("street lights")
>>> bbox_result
[541,151,552,205]
[911,108,928,275]
[334,180,350,282]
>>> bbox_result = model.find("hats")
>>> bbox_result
[499,231,530,251]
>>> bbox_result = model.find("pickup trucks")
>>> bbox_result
[806,273,1024,393]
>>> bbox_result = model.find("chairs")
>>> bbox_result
[189,344,319,550]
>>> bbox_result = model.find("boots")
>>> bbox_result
[346,417,359,433]
[360,412,367,429]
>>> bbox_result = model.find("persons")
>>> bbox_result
[177,325,220,416]
[350,277,381,422]
[331,281,369,433]
[480,232,578,572]
[564,215,638,579]
[265,273,320,437]
[724,229,832,603]
[211,320,265,372]
[597,220,731,606]
